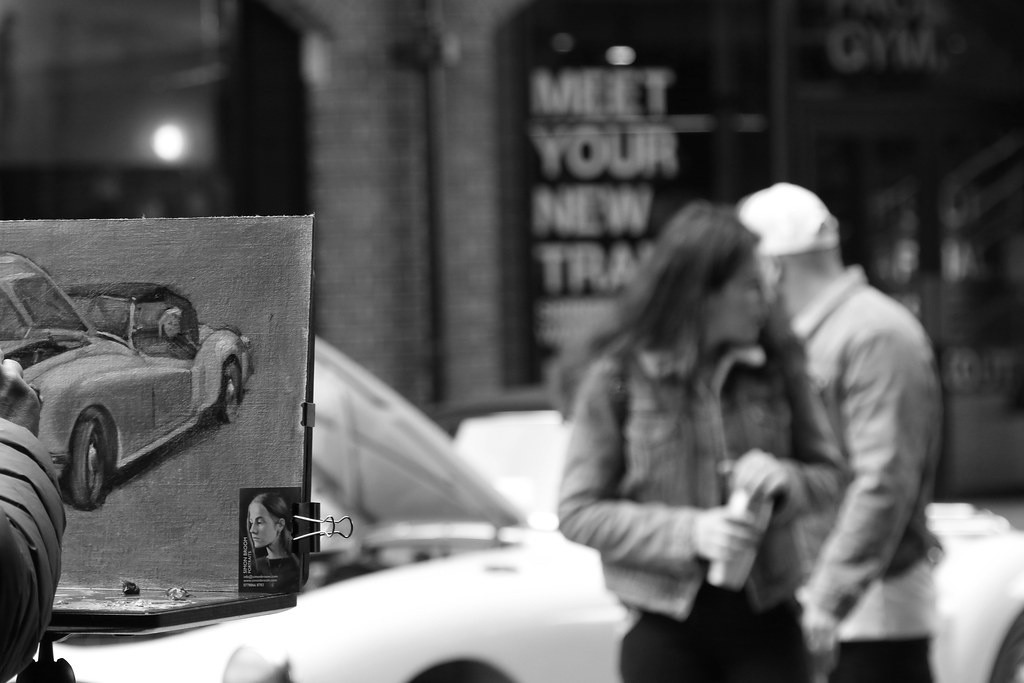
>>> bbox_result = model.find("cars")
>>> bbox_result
[36,335,1024,683]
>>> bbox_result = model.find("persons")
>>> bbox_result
[0,349,67,683]
[553,204,850,683]
[736,182,945,683]
[245,492,300,592]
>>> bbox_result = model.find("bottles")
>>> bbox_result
[707,489,774,590]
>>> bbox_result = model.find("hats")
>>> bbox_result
[735,182,838,256]
